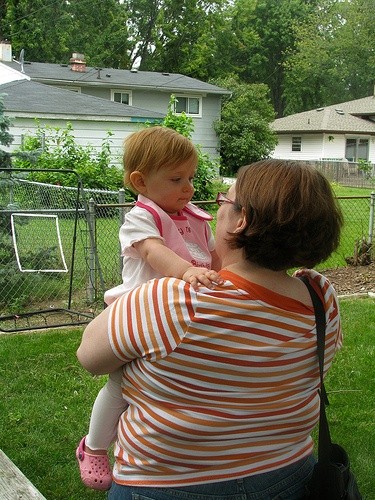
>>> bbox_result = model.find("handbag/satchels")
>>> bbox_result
[308,443,362,500]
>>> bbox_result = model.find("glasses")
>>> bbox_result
[217,193,235,208]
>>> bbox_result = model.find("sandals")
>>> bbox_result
[75,435,113,490]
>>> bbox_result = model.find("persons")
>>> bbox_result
[75,157,344,500]
[76,127,222,491]
